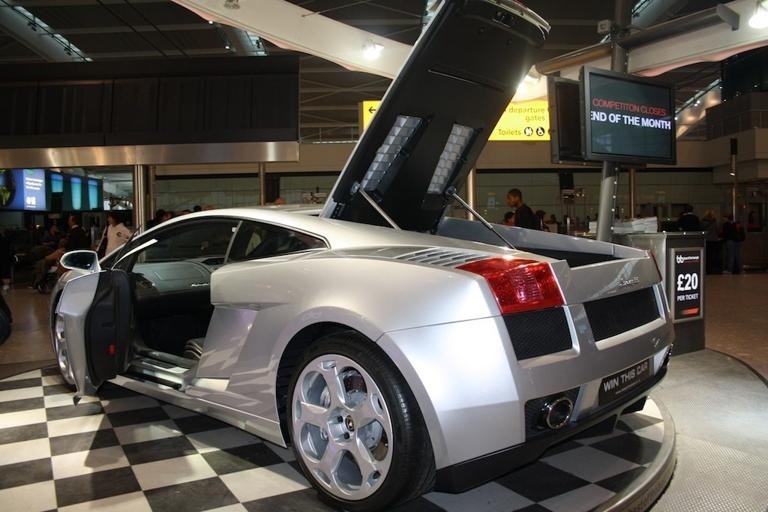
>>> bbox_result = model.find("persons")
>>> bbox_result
[27,203,214,290]
[546,214,560,225]
[506,188,540,229]
[273,198,286,205]
[536,210,549,231]
[560,214,572,224]
[678,204,757,275]
[503,212,515,226]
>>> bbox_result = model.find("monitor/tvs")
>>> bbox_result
[580,65,677,165]
[547,76,647,168]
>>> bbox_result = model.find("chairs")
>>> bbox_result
[183,232,290,360]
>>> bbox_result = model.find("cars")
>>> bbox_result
[48,0,677,512]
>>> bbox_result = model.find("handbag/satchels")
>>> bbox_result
[98,225,108,260]
